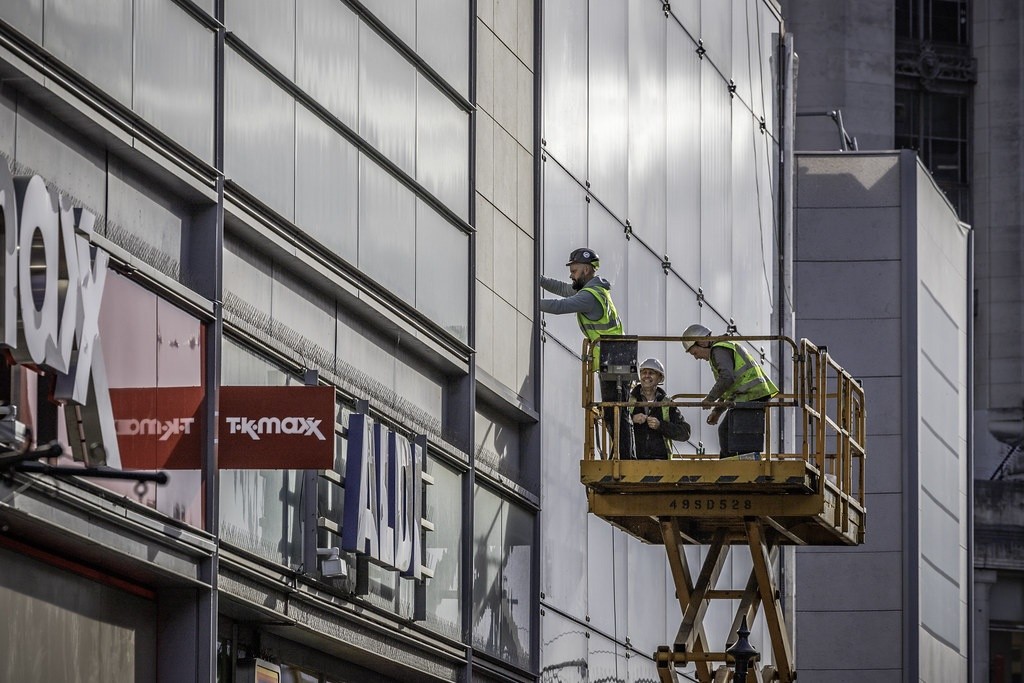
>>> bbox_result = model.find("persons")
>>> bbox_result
[538,247,636,460]
[682,324,779,458]
[629,358,691,460]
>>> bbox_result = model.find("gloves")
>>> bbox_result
[647,416,659,429]
[632,413,648,424]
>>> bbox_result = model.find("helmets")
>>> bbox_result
[681,325,712,353]
[565,248,600,272]
[639,358,665,384]
